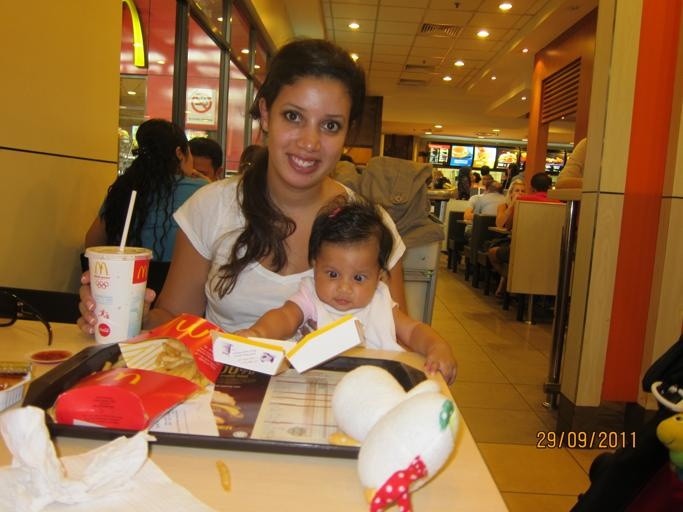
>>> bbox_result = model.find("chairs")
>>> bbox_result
[463,214,495,296]
[447,211,465,272]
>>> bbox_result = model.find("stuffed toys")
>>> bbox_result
[655,414,683,480]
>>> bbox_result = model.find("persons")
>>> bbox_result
[85,118,212,293]
[465,180,505,240]
[489,171,561,277]
[495,178,525,299]
[78,38,407,339]
[556,137,588,188]
[481,166,493,184]
[234,202,457,386]
[434,171,451,189]
[464,182,490,219]
[188,136,224,182]
[471,173,485,188]
[417,152,432,211]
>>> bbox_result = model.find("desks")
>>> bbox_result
[427,189,458,219]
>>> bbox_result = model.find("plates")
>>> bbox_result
[30,350,72,363]
[1,362,36,412]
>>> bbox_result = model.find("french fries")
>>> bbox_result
[216,460,230,492]
[153,340,212,400]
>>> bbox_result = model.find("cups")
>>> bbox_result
[85,246,151,344]
